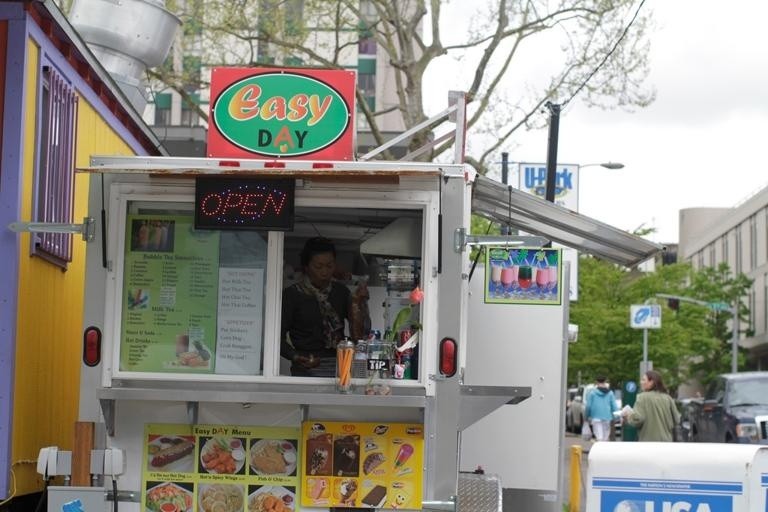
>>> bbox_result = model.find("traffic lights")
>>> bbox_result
[668,298,679,309]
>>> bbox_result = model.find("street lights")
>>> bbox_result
[501,152,624,235]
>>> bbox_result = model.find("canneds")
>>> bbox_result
[367,329,381,354]
[390,330,418,379]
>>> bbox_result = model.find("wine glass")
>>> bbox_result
[490,259,558,301]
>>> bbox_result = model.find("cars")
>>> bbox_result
[566,371,768,444]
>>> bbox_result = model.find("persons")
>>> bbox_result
[279,235,373,377]
[582,375,619,442]
[621,371,681,443]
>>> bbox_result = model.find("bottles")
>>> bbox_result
[334,337,354,396]
[365,341,394,396]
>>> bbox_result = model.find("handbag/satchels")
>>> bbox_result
[581,420,593,442]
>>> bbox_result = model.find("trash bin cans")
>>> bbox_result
[586,442,768,512]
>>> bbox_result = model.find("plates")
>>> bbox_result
[147,435,195,473]
[248,485,296,512]
[197,483,245,512]
[200,436,246,475]
[249,439,297,476]
[146,481,194,512]
